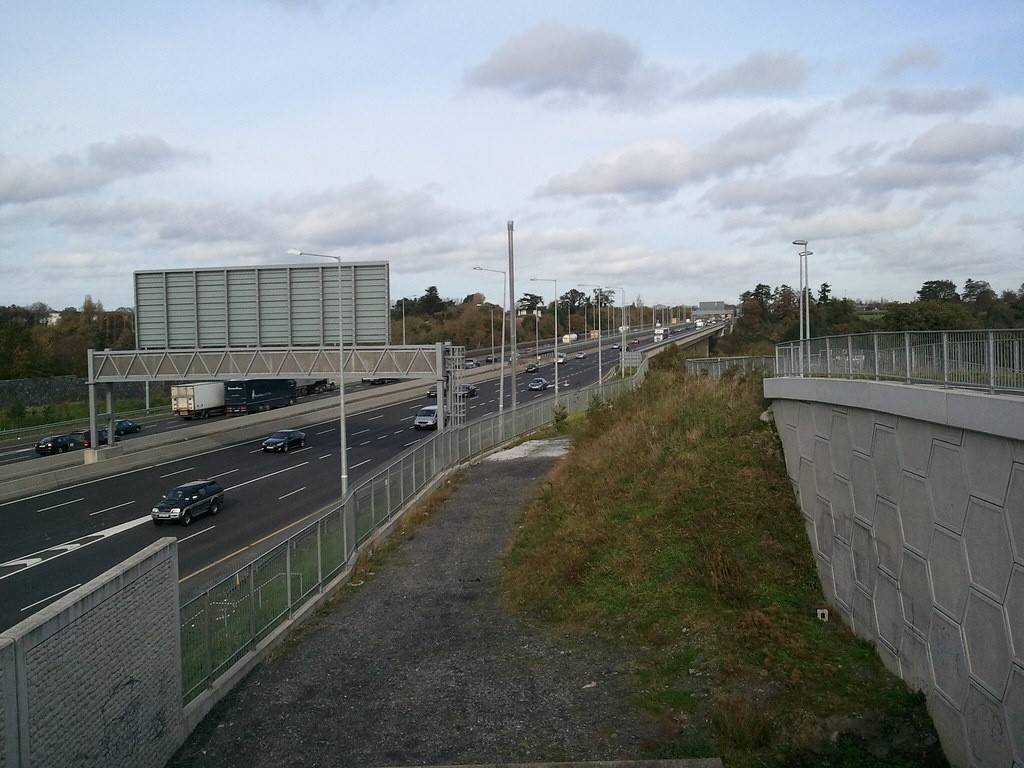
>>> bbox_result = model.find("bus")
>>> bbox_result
[653,328,668,342]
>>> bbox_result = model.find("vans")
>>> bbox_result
[413,406,450,430]
[553,353,567,364]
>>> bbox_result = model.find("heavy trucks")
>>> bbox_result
[562,333,580,344]
[223,379,296,415]
[171,377,337,420]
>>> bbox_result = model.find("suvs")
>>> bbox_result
[151,478,225,527]
[66,429,121,451]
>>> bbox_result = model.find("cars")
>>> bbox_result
[836,345,867,369]
[452,384,478,398]
[632,338,640,344]
[427,384,448,397]
[262,430,306,452]
[464,359,481,368]
[486,355,497,363]
[575,351,586,359]
[104,419,142,436]
[362,378,400,384]
[526,363,539,373]
[34,435,71,455]
[619,344,630,351]
[612,343,620,349]
[669,325,691,334]
[528,377,548,391]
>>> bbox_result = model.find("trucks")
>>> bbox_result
[620,325,630,334]
[590,330,602,340]
[696,322,706,331]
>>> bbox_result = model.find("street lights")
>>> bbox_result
[403,295,418,345]
[578,284,601,394]
[530,277,559,402]
[472,266,506,440]
[605,286,626,377]
[793,240,812,378]
[799,251,815,378]
[286,248,349,500]
